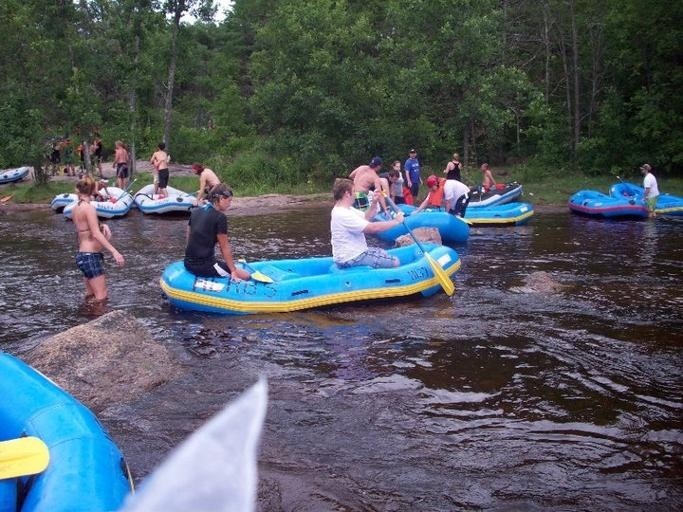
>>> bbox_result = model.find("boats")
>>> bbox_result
[154,246,468,312]
[132,180,200,216]
[53,194,69,210]
[573,187,647,220]
[456,183,527,206]
[0,165,29,183]
[372,203,469,242]
[1,342,144,512]
[457,202,536,224]
[64,183,135,219]
[614,184,683,219]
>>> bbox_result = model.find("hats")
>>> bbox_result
[369,156,382,168]
[409,149,416,153]
[639,164,651,172]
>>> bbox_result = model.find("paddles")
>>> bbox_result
[381,188,454,297]
[238,258,273,284]
[0,435,49,479]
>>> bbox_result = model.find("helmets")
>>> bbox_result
[426,175,438,188]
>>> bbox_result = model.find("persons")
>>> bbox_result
[330,177,406,269]
[182,182,252,284]
[151,152,160,195]
[639,163,660,220]
[71,174,126,301]
[444,153,463,181]
[41,130,132,202]
[410,174,472,219]
[470,163,505,195]
[150,142,169,198]
[191,163,221,209]
[349,145,422,221]
